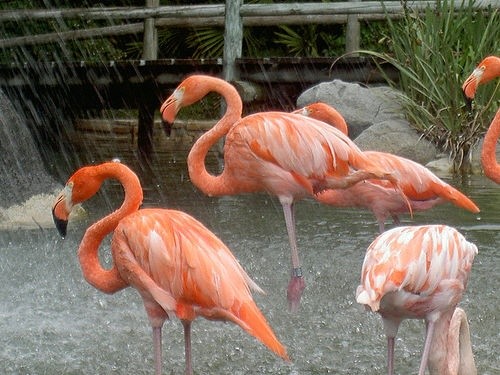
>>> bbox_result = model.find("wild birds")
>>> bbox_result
[50,162,290,375]
[291,100,481,233]
[459,57,500,184]
[157,73,413,315]
[352,221,480,375]
